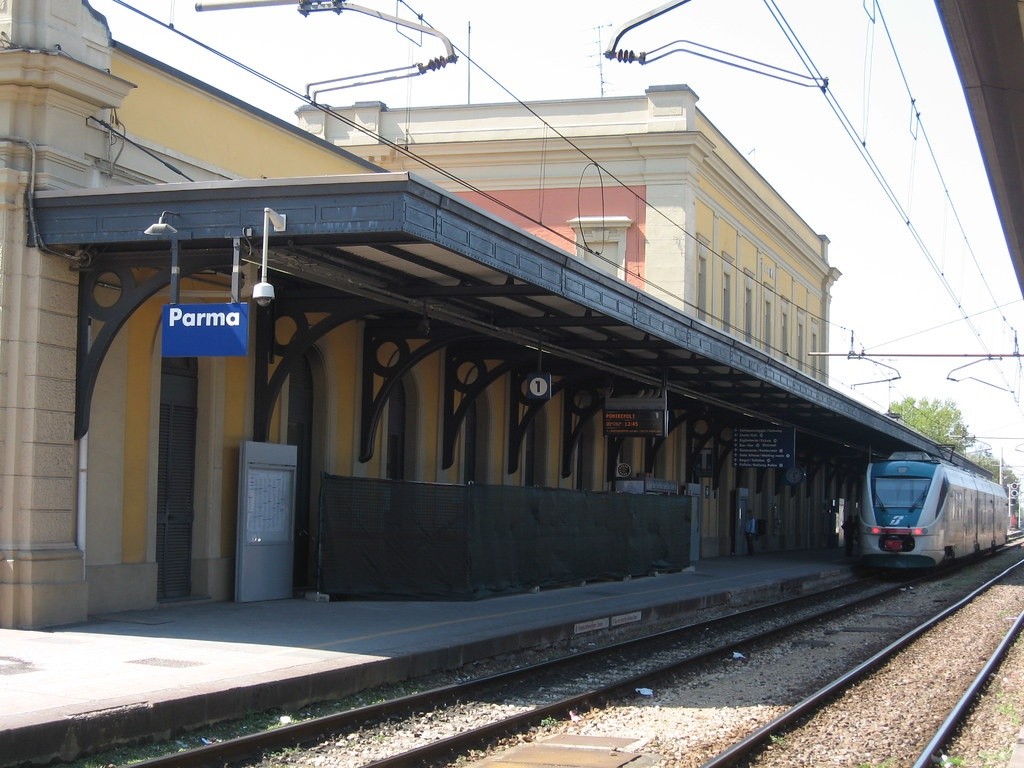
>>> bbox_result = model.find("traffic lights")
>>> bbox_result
[1011,483,1018,497]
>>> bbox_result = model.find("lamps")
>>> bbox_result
[143,211,181,236]
[616,462,632,479]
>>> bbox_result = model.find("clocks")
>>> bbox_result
[785,465,803,485]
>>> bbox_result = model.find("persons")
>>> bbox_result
[745,509,756,554]
[842,515,855,557]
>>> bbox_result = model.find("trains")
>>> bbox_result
[858,451,1009,569]
